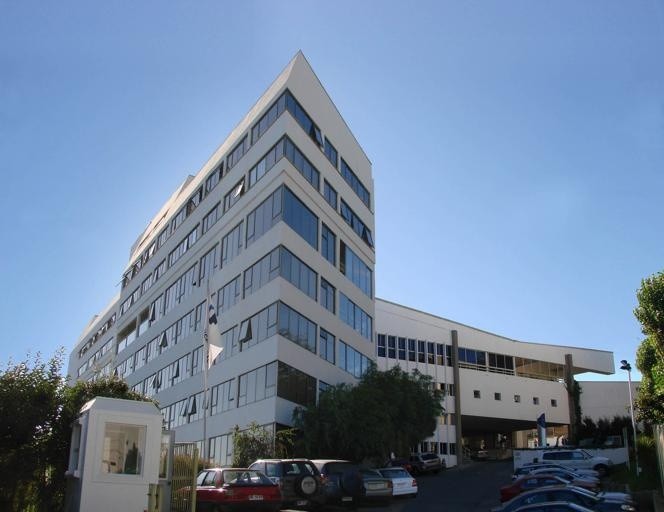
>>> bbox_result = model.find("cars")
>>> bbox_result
[490,449,638,512]
[176,458,418,512]
[413,453,442,473]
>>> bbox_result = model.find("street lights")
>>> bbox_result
[620,360,639,477]
